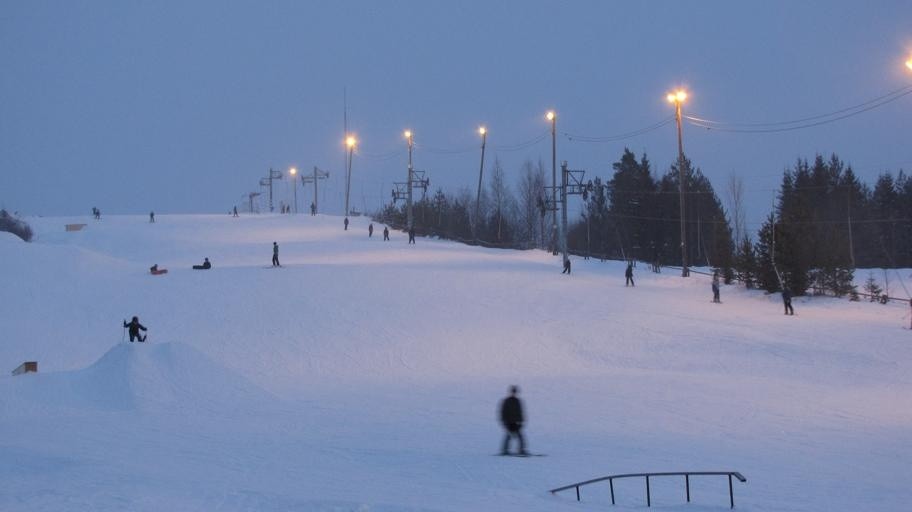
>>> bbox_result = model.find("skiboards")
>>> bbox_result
[710,300,724,304]
[138,335,147,341]
[782,313,798,316]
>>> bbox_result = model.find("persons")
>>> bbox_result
[234,205,238,216]
[384,227,390,241]
[150,210,155,223]
[95,209,100,218]
[192,257,211,270]
[624,264,634,287]
[562,254,571,273]
[369,223,373,236]
[122,316,148,342]
[311,202,316,216]
[780,283,794,316]
[498,382,527,456]
[344,217,349,230]
[271,242,280,266]
[408,226,415,243]
[712,273,720,302]
[149,263,168,275]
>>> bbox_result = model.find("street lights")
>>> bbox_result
[404,130,415,233]
[545,109,559,259]
[344,133,356,217]
[289,167,297,214]
[667,88,694,278]
[472,125,488,243]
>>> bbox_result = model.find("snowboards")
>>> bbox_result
[488,452,546,458]
